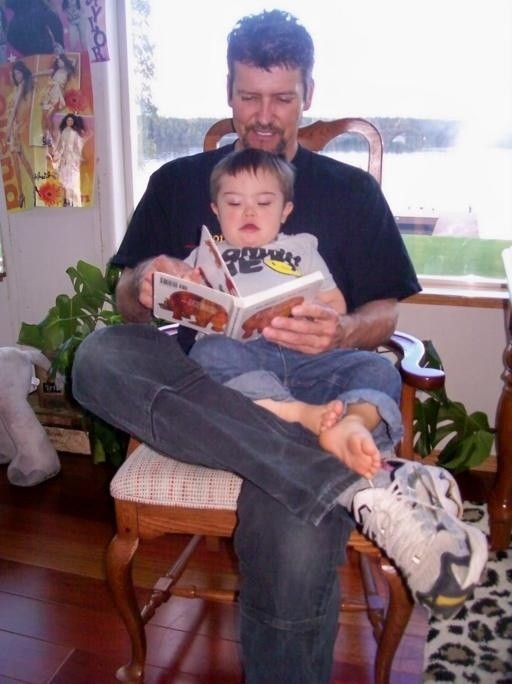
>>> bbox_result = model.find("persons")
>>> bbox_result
[6,54,93,208]
[181,147,404,481]
[69,6,490,683]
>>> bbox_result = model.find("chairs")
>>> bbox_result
[99,114,447,684]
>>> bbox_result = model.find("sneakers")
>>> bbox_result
[352,457,489,621]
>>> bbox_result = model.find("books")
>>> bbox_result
[154,224,325,343]
